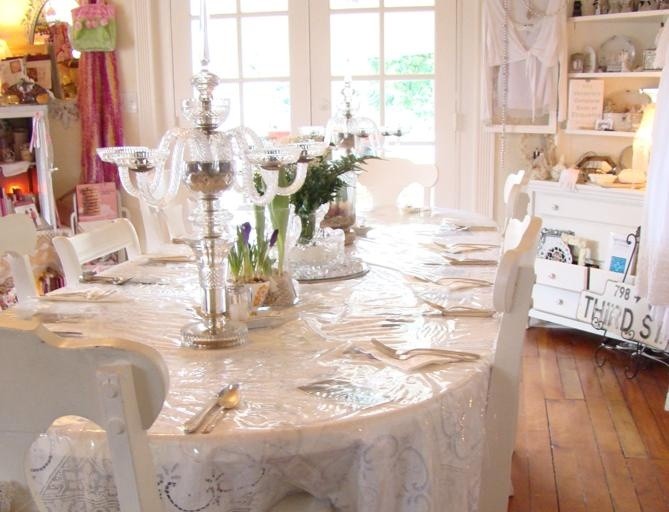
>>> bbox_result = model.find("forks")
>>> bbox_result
[424,300,497,312]
[424,275,493,285]
[371,338,480,358]
[434,242,499,248]
[442,254,497,262]
[78,276,133,284]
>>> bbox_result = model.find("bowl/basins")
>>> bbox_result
[602,112,628,131]
[588,174,618,184]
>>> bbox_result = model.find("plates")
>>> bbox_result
[598,35,635,71]
[538,240,572,263]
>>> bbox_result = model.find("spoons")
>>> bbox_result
[375,346,476,361]
[443,248,492,254]
[429,305,494,317]
[445,259,497,265]
[406,273,495,285]
[201,388,241,434]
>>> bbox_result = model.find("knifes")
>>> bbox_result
[184,383,239,434]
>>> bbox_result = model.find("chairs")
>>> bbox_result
[474,172,543,512]
[51,216,138,287]
[0,212,38,304]
[0,314,170,512]
[352,155,440,205]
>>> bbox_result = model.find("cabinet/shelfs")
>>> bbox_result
[1,99,84,230]
[523,0,669,354]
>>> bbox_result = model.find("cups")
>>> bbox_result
[13,128,27,159]
[225,285,253,322]
